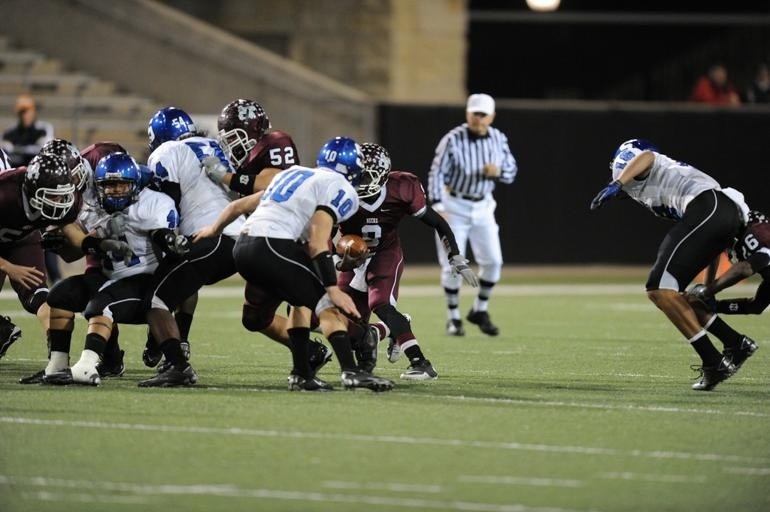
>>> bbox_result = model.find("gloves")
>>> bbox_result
[446,253,481,289]
[162,231,192,256]
[104,210,136,237]
[202,153,227,182]
[588,178,623,213]
[100,238,134,266]
[337,246,376,273]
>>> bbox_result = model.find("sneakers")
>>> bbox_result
[689,355,737,391]
[285,369,334,392]
[466,307,501,337]
[400,359,439,381]
[0,316,22,357]
[18,369,45,384]
[141,325,162,368]
[357,326,380,373]
[155,340,191,375]
[340,371,394,392]
[721,336,758,368]
[70,362,101,385]
[385,312,412,362]
[42,371,73,386]
[138,364,199,387]
[96,349,126,377]
[306,337,333,375]
[445,317,463,336]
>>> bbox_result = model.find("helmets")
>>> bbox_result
[38,137,88,190]
[146,105,196,152]
[216,98,271,169]
[24,154,76,220]
[614,138,657,157]
[316,135,365,181]
[726,208,768,265]
[360,141,391,198]
[92,151,141,214]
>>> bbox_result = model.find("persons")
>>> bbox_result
[196,96,298,190]
[2,96,55,166]
[428,94,517,337]
[16,151,189,387]
[191,136,396,394]
[139,106,245,388]
[695,64,738,105]
[746,65,769,100]
[1,157,134,356]
[338,142,477,382]
[592,137,758,391]
[688,210,770,317]
[38,137,164,378]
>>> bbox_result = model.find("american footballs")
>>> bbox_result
[337,235,367,259]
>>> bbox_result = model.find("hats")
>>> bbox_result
[15,95,34,111]
[466,92,497,116]
